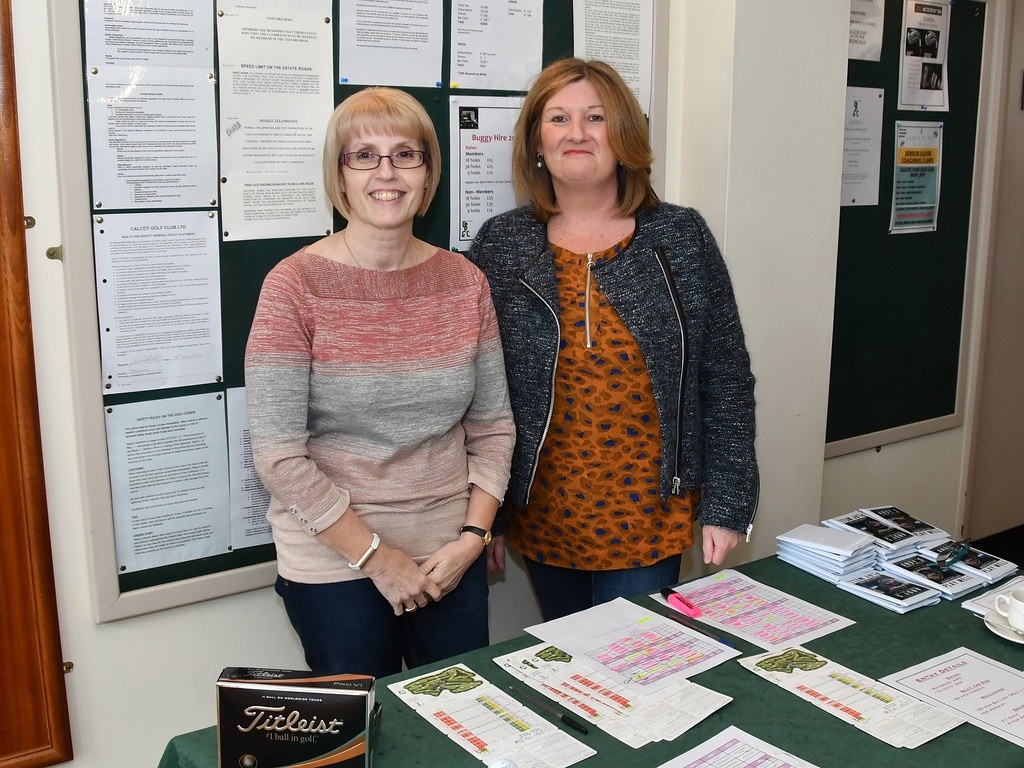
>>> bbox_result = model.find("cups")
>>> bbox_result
[995,588,1024,632]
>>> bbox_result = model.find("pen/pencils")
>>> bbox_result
[508,685,589,734]
[671,614,736,650]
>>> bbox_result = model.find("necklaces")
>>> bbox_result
[344,229,419,270]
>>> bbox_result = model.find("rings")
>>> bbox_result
[405,605,416,612]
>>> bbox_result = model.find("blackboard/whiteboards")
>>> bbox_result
[52,2,670,626]
[825,1,987,461]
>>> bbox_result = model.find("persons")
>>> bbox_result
[468,58,760,623]
[245,87,516,679]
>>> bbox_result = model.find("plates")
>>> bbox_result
[984,605,1024,644]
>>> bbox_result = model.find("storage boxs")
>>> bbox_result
[215,666,384,768]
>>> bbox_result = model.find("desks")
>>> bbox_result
[156,553,1024,768]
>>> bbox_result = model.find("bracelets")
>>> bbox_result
[348,533,380,570]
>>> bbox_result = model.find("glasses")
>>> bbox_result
[339,150,427,171]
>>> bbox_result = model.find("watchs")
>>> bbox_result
[460,526,492,545]
